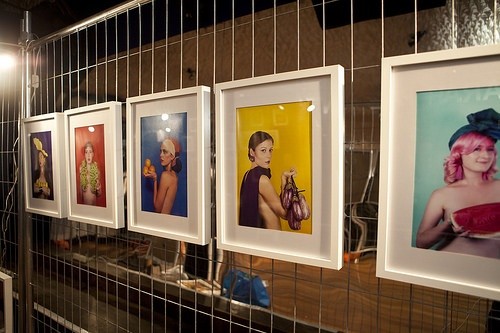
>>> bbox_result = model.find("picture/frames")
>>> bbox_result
[214,65,345,272]
[64,101,125,229]
[125,85,212,246]
[20,112,67,219]
[375,44,500,300]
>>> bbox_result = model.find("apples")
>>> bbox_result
[143,159,155,174]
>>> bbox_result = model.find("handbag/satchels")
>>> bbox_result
[222,269,270,308]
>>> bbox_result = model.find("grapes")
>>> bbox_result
[81,160,98,193]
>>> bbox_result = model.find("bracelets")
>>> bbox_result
[153,178,158,182]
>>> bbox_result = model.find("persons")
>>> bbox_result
[239,131,296,230]
[79,143,102,206]
[416,108,500,259]
[143,137,181,214]
[32,150,50,200]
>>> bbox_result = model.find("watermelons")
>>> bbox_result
[450,202,500,238]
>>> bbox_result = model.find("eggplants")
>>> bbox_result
[282,183,310,230]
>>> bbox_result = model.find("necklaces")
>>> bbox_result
[81,160,99,193]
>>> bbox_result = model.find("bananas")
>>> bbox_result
[38,181,48,188]
